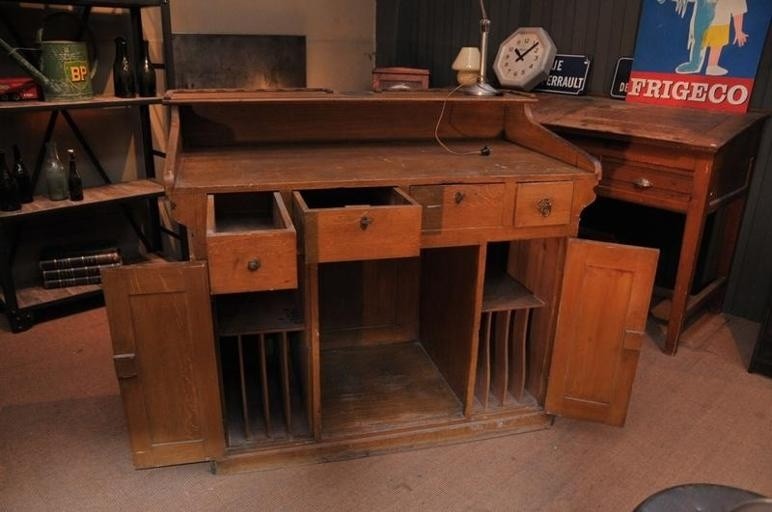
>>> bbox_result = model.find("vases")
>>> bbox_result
[113,39,156,98]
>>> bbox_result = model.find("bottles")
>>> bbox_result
[114,37,156,98]
[1,144,32,213]
[42,140,83,200]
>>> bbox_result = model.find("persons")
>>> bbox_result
[675,0,752,77]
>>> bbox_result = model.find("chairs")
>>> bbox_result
[372,67,430,92]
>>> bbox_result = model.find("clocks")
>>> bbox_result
[493,27,558,91]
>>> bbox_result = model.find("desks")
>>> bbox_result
[532,83,772,356]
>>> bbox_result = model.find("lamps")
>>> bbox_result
[452,47,482,86]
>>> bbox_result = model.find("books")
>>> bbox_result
[40,247,123,288]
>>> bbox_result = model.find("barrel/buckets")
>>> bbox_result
[38,41,95,100]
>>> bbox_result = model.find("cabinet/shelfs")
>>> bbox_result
[0,0,175,336]
[416,87,660,450]
[317,244,467,464]
[293,86,484,266]
[104,85,323,476]
